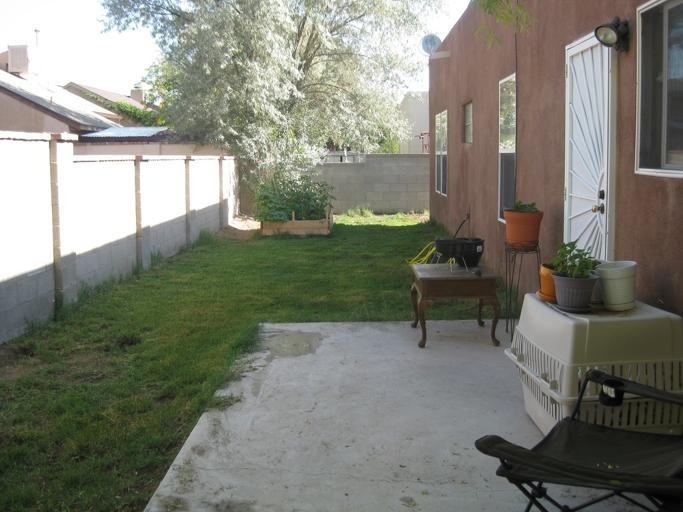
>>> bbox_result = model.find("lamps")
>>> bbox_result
[593,14,631,53]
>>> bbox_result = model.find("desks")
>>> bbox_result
[407,260,503,351]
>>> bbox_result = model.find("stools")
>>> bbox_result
[471,365,681,511]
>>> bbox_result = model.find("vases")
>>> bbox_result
[587,257,604,305]
[534,261,558,301]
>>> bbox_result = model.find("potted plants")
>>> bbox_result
[500,199,546,251]
[549,240,599,308]
